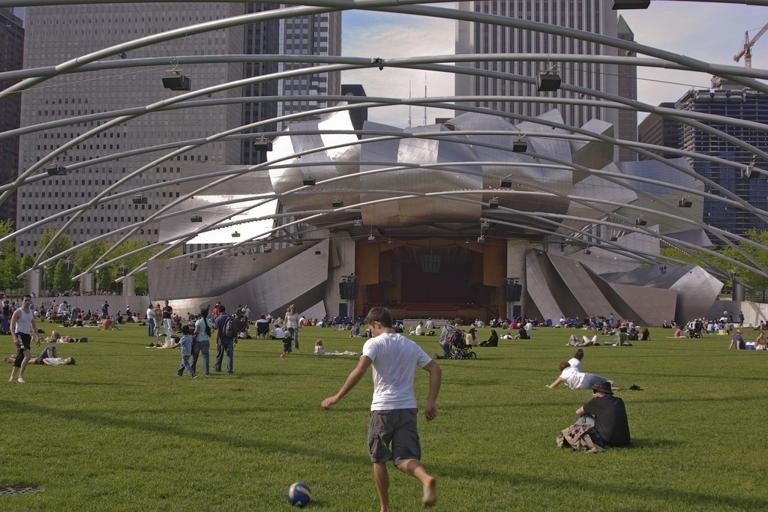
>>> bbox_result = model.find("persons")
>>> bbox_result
[171,324,196,379]
[185,302,252,341]
[319,304,442,512]
[1,294,110,343]
[574,379,632,453]
[8,357,75,366]
[255,304,319,356]
[557,313,651,347]
[544,361,621,390]
[190,308,213,377]
[448,316,554,347]
[395,317,435,336]
[212,305,239,376]
[117,299,181,340]
[322,312,372,338]
[568,348,584,371]
[663,310,767,351]
[6,293,36,386]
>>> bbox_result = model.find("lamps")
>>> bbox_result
[478,60,562,248]
[581,153,760,256]
[160,59,193,94]
[45,134,344,239]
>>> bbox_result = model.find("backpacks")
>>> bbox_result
[222,316,236,337]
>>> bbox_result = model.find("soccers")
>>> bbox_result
[286,481,310,507]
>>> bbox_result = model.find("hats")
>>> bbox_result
[592,381,611,394]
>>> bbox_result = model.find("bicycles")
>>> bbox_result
[446,337,477,360]
